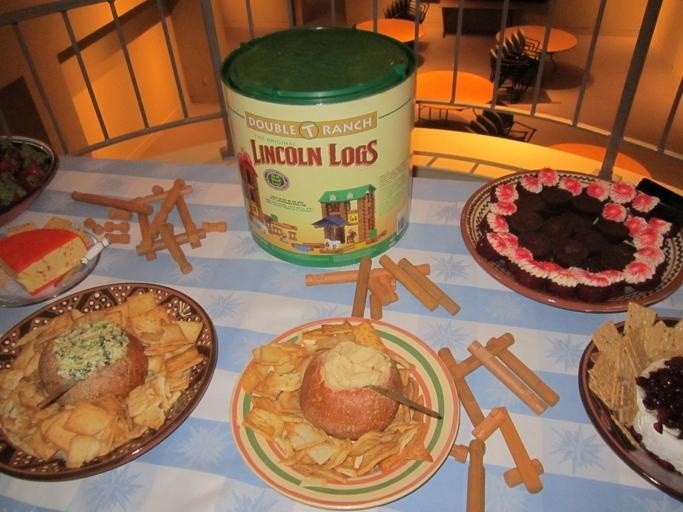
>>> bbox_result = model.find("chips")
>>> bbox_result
[588,302,683,454]
[0,292,203,468]
[240,320,433,486]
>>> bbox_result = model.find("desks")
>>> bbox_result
[414,70,493,124]
[495,25,577,73]
[550,144,652,180]
[357,18,424,44]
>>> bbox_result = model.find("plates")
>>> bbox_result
[578,317,681,505]
[0,282,218,483]
[459,170,683,315]
[4,224,100,311]
[231,317,463,512]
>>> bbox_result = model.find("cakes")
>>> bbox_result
[477,169,679,302]
[1,229,87,296]
[630,355,683,478]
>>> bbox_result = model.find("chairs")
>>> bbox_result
[488,27,538,90]
[465,101,535,142]
[383,0,429,24]
[487,28,544,102]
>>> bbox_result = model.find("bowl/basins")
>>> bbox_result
[1,130,61,228]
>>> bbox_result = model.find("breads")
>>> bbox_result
[39,320,148,405]
[299,341,403,438]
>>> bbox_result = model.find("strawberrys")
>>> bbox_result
[1,135,46,211]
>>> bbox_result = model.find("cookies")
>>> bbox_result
[433,332,560,512]
[306,252,459,320]
[72,179,229,274]
